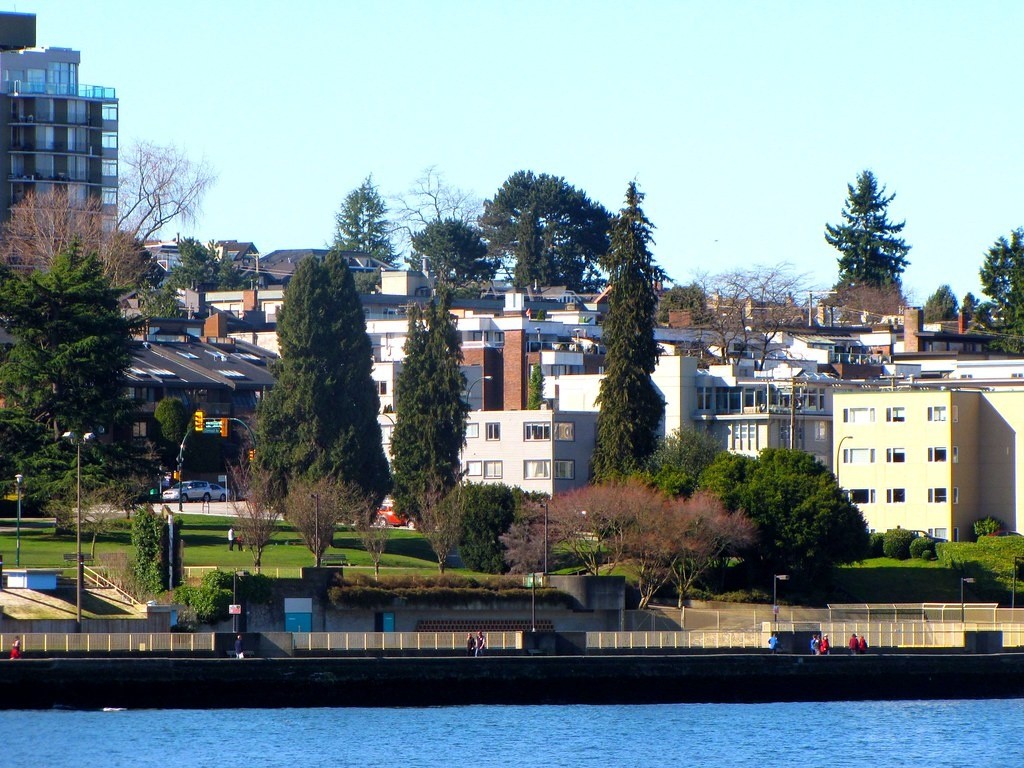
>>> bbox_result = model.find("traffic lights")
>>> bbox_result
[194,412,202,431]
[220,421,226,435]
[249,450,254,462]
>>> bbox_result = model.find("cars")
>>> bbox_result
[209,483,232,502]
[987,530,1024,536]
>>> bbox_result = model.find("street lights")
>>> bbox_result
[15,474,23,567]
[531,571,548,632]
[535,502,549,573]
[232,569,251,632]
[774,573,789,621]
[309,492,320,567]
[960,576,976,622]
[63,431,96,633]
[836,435,853,487]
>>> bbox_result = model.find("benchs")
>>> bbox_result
[63,554,94,565]
[319,553,348,565]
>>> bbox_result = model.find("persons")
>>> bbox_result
[767,633,778,654]
[236,534,244,551]
[809,634,830,655]
[848,634,858,654]
[234,634,244,659]
[9,635,22,659]
[227,526,235,551]
[466,632,476,656]
[474,630,486,658]
[858,634,867,654]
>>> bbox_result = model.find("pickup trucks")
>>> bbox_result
[909,530,948,544]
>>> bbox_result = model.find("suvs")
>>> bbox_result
[162,480,213,502]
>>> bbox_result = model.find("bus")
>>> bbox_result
[374,493,418,529]
